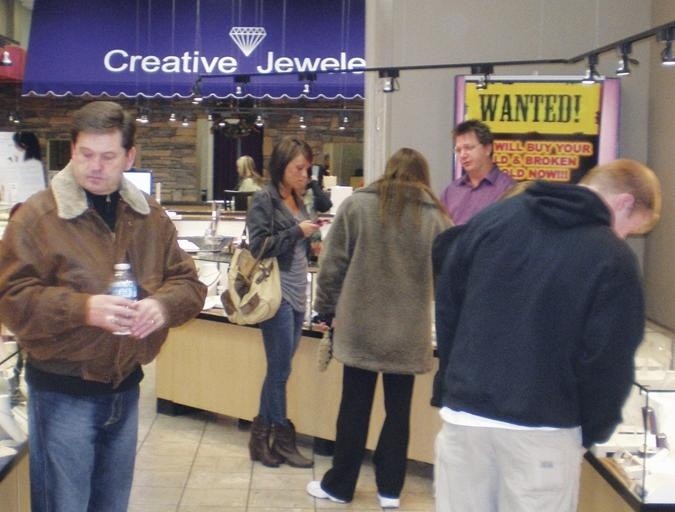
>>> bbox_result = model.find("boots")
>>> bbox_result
[248,413,286,468]
[271,422,315,470]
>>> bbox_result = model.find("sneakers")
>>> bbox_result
[306,479,347,504]
[375,489,400,509]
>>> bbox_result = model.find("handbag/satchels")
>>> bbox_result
[220,189,282,327]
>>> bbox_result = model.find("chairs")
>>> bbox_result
[223,190,255,211]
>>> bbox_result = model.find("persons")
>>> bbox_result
[431,159,661,512]
[245,138,320,468]
[233,155,267,210]
[438,120,516,226]
[307,147,452,508]
[296,168,332,212]
[0,100,207,512]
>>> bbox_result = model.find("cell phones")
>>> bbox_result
[311,213,334,225]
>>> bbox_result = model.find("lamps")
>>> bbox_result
[132,106,353,131]
[192,58,495,102]
[582,25,675,83]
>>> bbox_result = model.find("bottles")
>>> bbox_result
[104,261,137,337]
[225,200,235,212]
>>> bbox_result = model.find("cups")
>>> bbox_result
[0,395,12,416]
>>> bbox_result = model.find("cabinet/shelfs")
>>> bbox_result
[0,317,32,511]
[148,235,674,512]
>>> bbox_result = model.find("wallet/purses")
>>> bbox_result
[315,328,334,375]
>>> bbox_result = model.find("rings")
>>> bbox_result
[112,318,117,324]
[150,320,156,324]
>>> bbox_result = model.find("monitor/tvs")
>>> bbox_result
[122,171,153,196]
